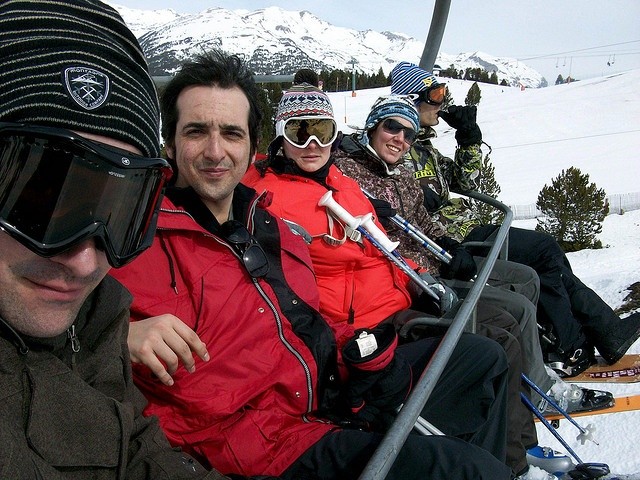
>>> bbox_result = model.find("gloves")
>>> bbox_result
[408,272,459,317]
[341,327,412,411]
[436,236,477,282]
[369,198,396,228]
[437,105,482,145]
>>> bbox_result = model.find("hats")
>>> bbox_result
[391,61,437,105]
[266,68,334,173]
[1,1,161,163]
[357,94,420,148]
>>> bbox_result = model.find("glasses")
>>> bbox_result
[382,119,418,145]
[0,124,172,269]
[275,115,338,149]
[406,83,447,105]
[219,220,270,278]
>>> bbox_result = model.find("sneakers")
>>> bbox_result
[534,382,615,418]
[597,313,640,365]
[525,446,573,475]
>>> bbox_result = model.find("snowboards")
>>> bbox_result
[563,354,640,383]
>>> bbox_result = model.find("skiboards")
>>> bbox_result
[533,394,640,429]
[558,463,640,480]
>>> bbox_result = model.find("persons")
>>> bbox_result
[239,68,541,466]
[329,90,588,474]
[386,59,640,381]
[1,0,223,477]
[106,45,521,480]
